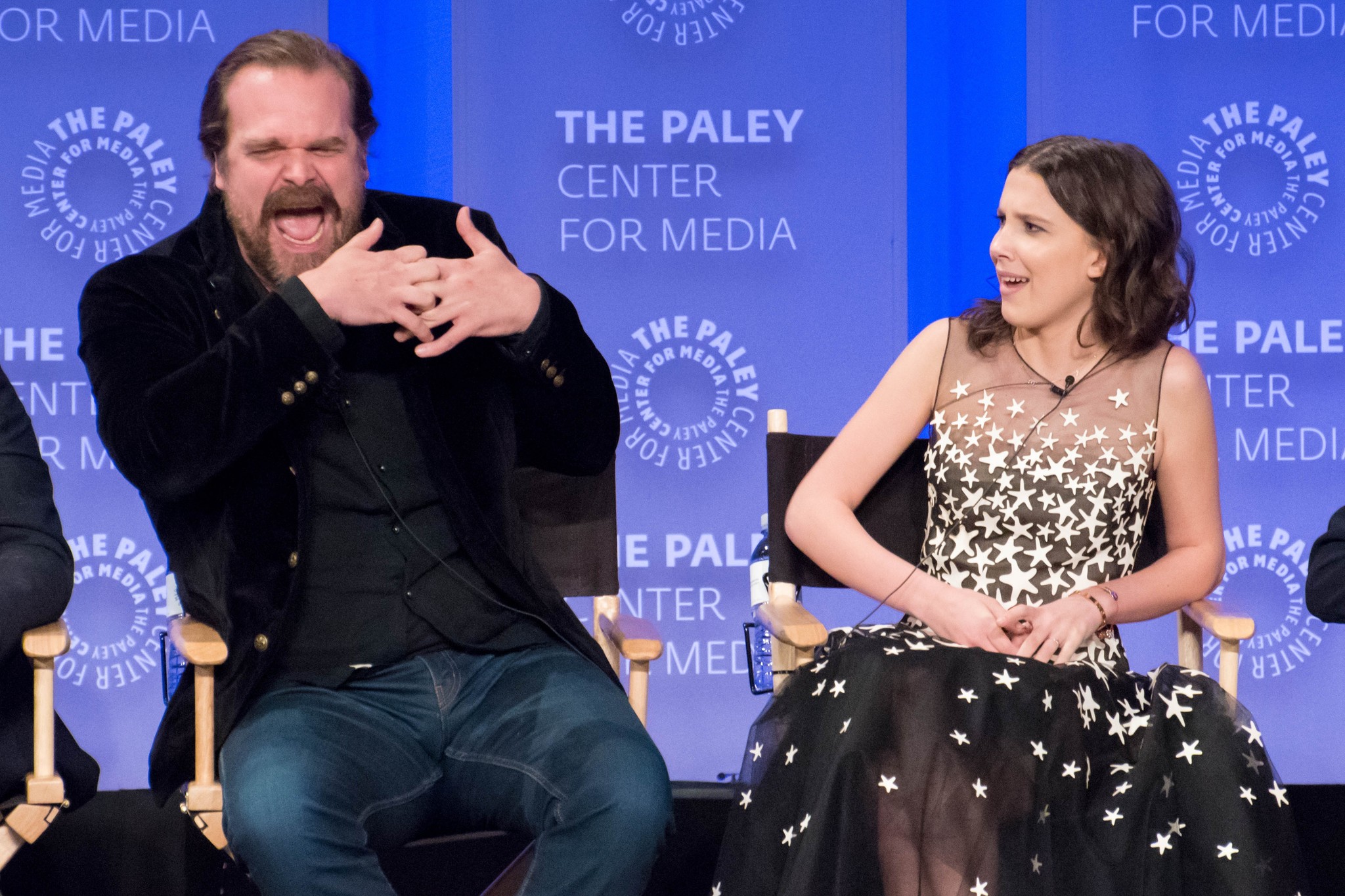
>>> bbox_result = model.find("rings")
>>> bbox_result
[1046,637,1060,648]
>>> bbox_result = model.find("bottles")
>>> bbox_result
[166,557,189,703]
[747,513,773,692]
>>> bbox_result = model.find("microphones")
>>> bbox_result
[1066,376,1075,388]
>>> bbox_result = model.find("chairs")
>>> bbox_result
[170,452,662,865]
[743,407,1255,721]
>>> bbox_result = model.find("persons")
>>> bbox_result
[0,372,104,820]
[78,29,680,896]
[707,137,1345,896]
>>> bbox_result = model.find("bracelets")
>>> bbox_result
[1068,589,1108,634]
[1093,586,1121,632]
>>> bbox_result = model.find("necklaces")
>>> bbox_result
[1014,328,1107,388]
